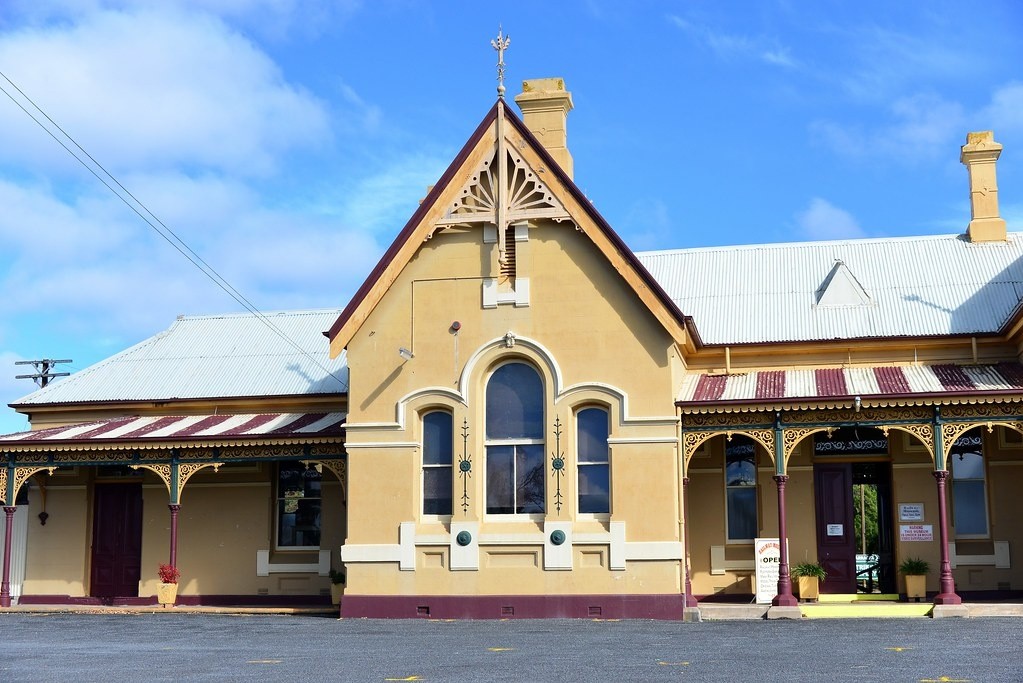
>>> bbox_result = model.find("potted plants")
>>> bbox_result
[329,570,345,604]
[896,553,935,597]
[791,560,828,598]
[156,562,180,604]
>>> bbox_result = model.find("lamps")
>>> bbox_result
[399,348,412,360]
